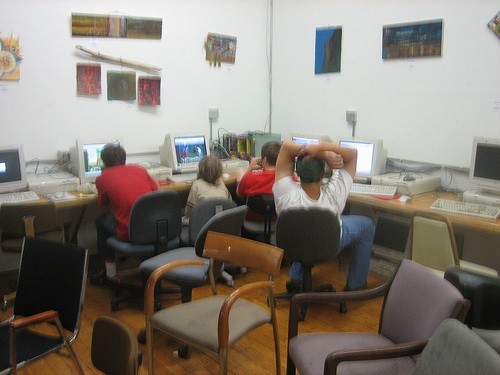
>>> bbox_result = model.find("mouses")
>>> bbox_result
[55,191,63,198]
[398,195,412,202]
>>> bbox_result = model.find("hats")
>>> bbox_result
[296,156,324,182]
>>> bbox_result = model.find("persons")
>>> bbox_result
[181,145,201,161]
[273,141,375,290]
[181,156,232,225]
[236,142,300,223]
[93,146,158,286]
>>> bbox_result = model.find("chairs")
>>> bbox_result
[0,190,500,375]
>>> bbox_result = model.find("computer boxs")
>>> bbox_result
[28,170,80,195]
[221,158,249,173]
[371,172,441,196]
[148,166,172,182]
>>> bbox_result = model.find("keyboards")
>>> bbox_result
[167,172,198,182]
[0,191,41,205]
[430,198,500,219]
[349,183,397,197]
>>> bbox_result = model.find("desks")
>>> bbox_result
[0,166,247,247]
[319,179,500,279]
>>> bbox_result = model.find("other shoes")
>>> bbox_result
[286,279,304,293]
[90,271,121,288]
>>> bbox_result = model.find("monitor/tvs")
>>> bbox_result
[0,144,30,193]
[70,135,122,186]
[469,137,500,197]
[289,133,335,145]
[338,137,388,184]
[159,132,211,174]
